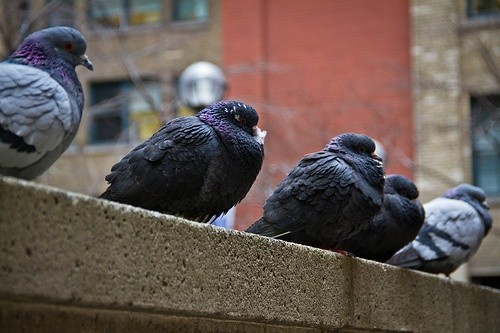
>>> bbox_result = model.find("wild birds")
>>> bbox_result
[245,131,386,257]
[328,174,426,264]
[0,26,94,181]
[97,99,265,225]
[386,182,494,288]
